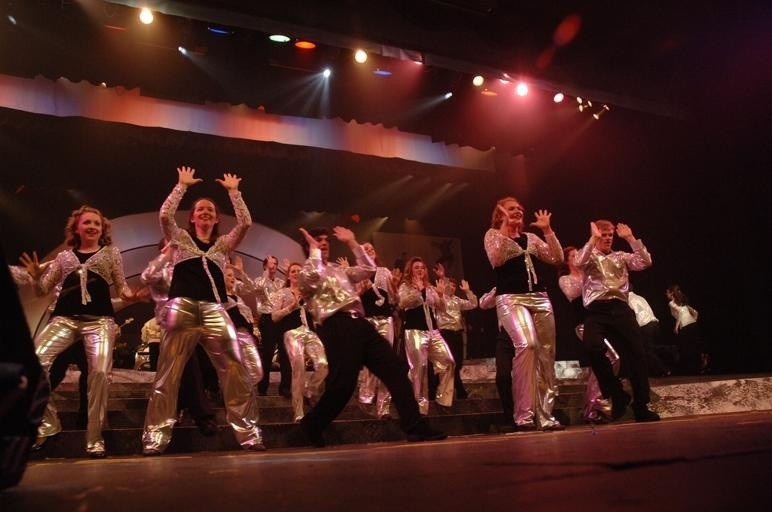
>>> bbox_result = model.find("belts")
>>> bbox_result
[335,311,363,318]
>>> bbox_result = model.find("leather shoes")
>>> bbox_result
[438,404,448,415]
[301,418,324,449]
[406,416,448,441]
[517,422,534,431]
[545,423,567,431]
[635,410,661,422]
[611,392,632,419]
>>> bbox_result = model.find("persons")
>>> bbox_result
[18,205,150,459]
[140,165,268,454]
[483,195,566,433]
[286,226,448,446]
[571,219,663,422]
[4,240,664,438]
[664,285,702,380]
